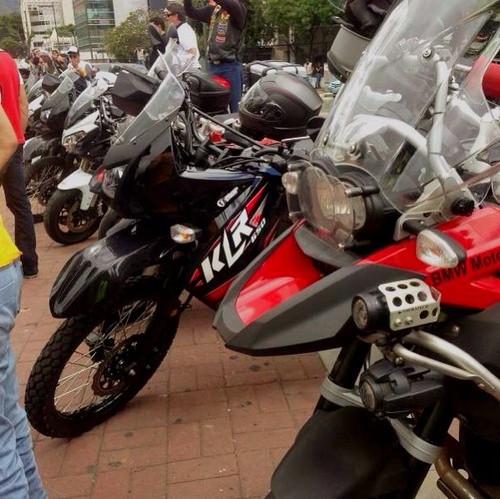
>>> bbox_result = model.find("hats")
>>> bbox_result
[67,46,79,54]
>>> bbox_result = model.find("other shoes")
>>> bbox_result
[23,266,39,279]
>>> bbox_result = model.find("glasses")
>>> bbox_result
[166,14,172,17]
[68,53,76,58]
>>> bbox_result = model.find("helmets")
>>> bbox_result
[237,71,324,142]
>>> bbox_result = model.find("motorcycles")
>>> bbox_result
[88,59,305,248]
[24,75,327,440]
[211,0,500,499]
[43,70,231,244]
[18,66,97,222]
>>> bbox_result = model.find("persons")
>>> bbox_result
[162,0,201,83]
[1,47,46,498]
[150,17,169,71]
[181,0,247,113]
[26,46,96,82]
